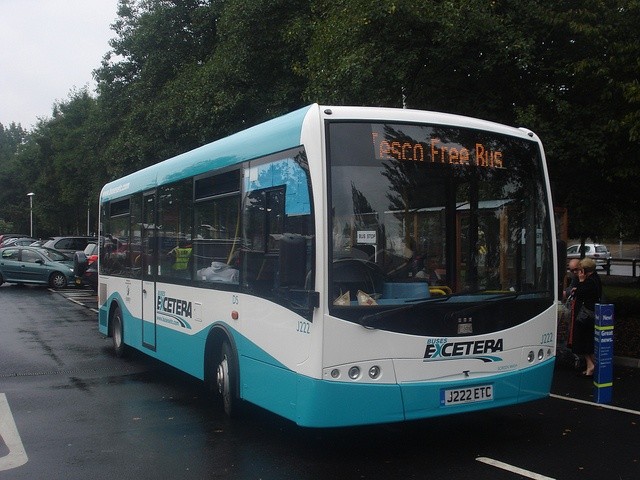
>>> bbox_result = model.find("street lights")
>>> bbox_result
[27,192,35,238]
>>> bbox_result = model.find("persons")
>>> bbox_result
[570,258,603,376]
[376,222,431,280]
[171,238,193,272]
[567,259,580,297]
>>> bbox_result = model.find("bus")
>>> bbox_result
[98,103,558,428]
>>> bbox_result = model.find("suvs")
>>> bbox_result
[567,244,611,270]
[73,241,98,294]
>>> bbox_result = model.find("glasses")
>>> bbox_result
[571,268,577,272]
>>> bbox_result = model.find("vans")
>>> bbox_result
[42,236,98,259]
[0,246,74,289]
